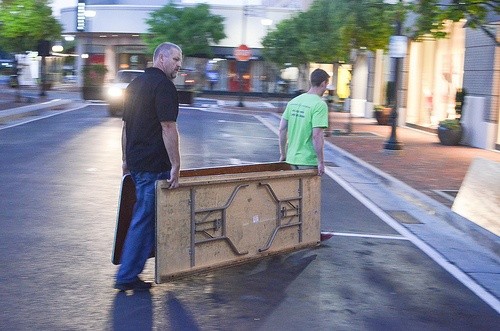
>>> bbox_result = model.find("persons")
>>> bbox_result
[112,42,183,290]
[279,68,333,241]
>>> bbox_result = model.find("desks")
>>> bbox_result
[112,161,292,263]
[155,169,320,284]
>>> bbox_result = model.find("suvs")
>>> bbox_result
[106,69,145,116]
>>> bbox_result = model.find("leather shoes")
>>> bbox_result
[114,274,155,291]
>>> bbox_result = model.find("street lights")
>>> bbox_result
[382,0,412,150]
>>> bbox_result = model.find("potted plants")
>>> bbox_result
[374,106,389,126]
[81,64,109,86]
[438,120,463,146]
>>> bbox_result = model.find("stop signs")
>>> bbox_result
[234,45,251,62]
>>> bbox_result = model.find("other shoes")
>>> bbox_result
[321,230,333,243]
